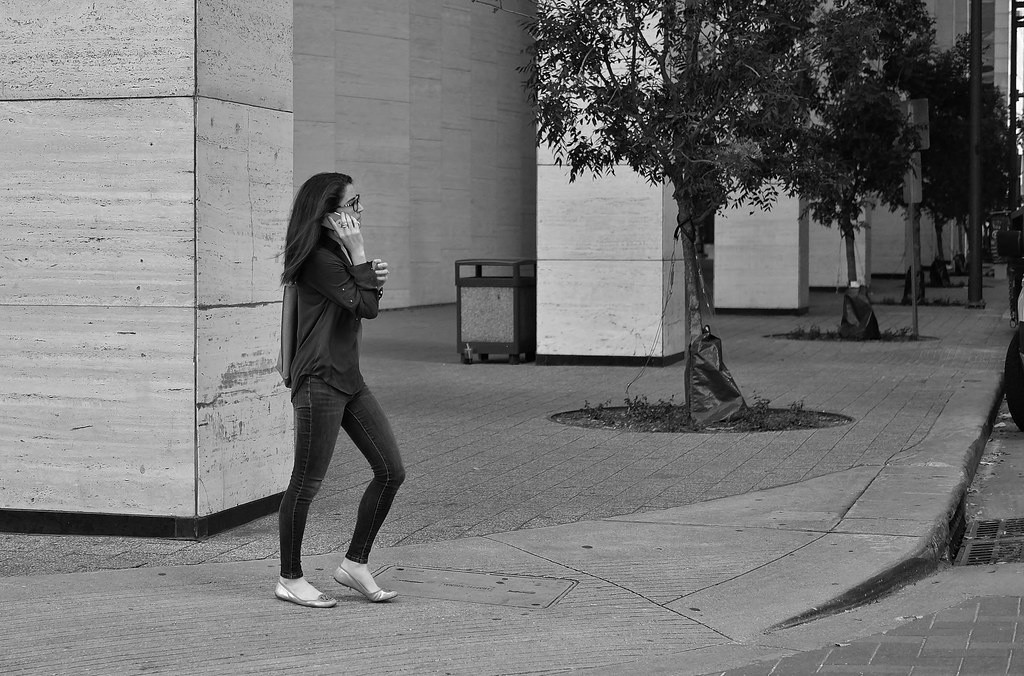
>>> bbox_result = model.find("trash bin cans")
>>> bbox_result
[454,258,537,365]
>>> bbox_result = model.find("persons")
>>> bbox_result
[276,171,406,609]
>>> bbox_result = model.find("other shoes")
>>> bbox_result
[275,580,338,608]
[334,566,398,602]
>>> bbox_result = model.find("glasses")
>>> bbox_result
[335,194,360,212]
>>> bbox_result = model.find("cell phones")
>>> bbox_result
[323,212,360,231]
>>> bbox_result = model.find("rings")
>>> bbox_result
[355,221,360,225]
[342,222,347,228]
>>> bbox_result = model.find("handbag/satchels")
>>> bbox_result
[274,282,297,388]
